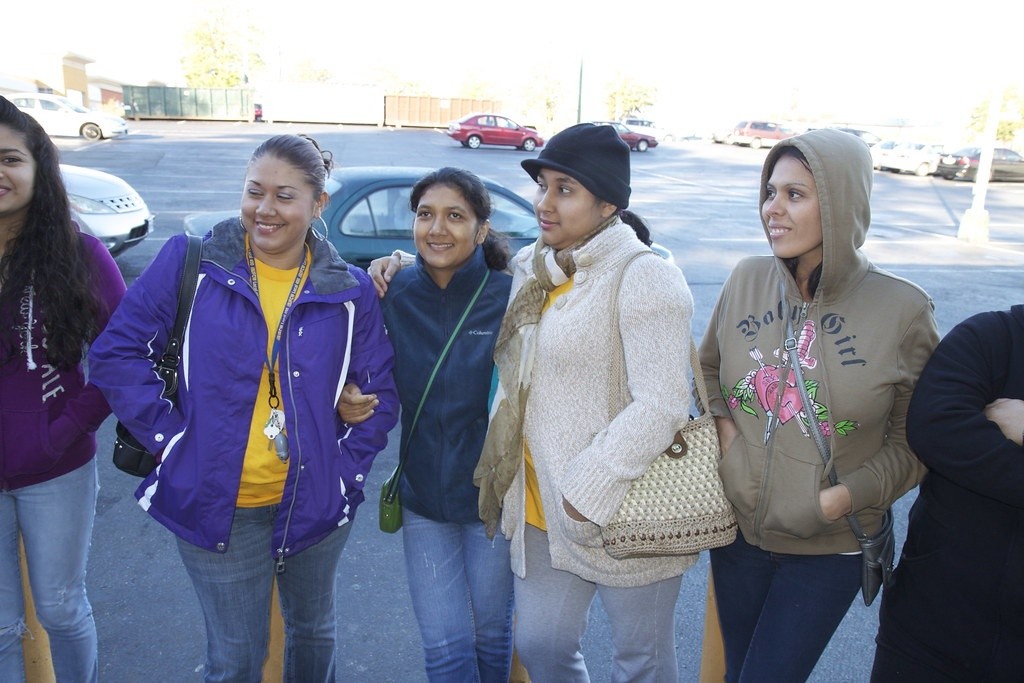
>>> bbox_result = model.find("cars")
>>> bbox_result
[622,116,677,145]
[184,162,545,264]
[57,162,157,259]
[442,113,545,154]
[711,117,941,177]
[591,122,658,153]
[931,140,1024,184]
[7,89,128,140]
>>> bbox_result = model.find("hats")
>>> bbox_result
[520,123,632,209]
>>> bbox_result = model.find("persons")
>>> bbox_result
[1,97,127,683]
[87,135,400,683]
[691,128,940,682]
[870,305,1024,683]
[367,123,693,683]
[337,168,512,682]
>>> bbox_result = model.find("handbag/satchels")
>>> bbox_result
[600,248,737,560]
[861,518,898,606]
[378,463,404,533]
[111,231,203,478]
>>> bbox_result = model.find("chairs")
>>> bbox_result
[393,194,414,230]
